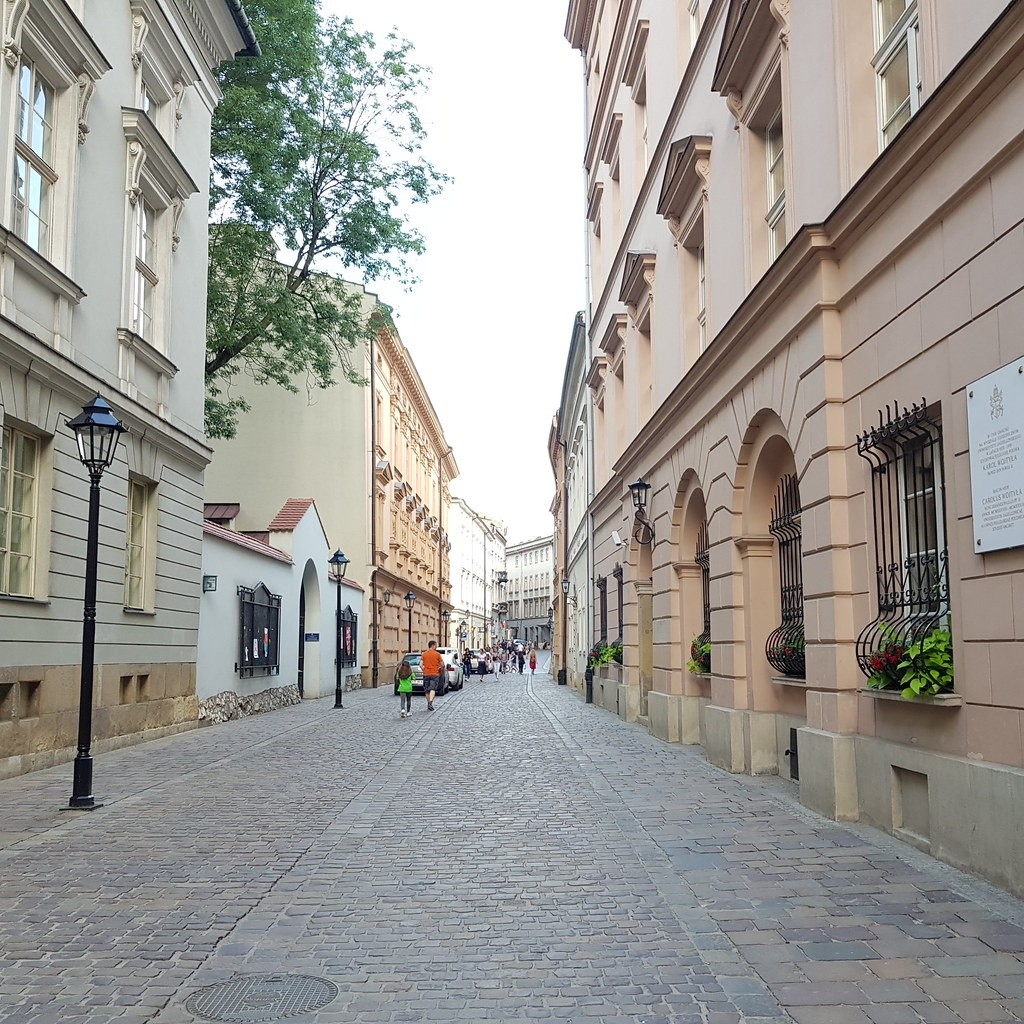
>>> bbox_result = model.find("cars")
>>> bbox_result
[463,649,495,674]
[394,646,466,695]
[513,638,529,654]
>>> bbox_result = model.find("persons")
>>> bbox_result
[396,661,416,718]
[462,647,473,682]
[477,639,537,683]
[419,640,445,711]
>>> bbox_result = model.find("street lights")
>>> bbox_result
[59,391,132,810]
[403,591,417,653]
[327,547,351,708]
[442,608,451,646]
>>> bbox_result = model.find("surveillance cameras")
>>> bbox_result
[612,531,622,547]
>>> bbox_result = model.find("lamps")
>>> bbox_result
[628,477,655,548]
[460,610,470,620]
[379,589,391,608]
[561,578,577,608]
[548,607,553,620]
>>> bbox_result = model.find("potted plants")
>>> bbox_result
[598,643,623,668]
[866,620,954,700]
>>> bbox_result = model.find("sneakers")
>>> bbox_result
[406,712,412,716]
[400,709,405,718]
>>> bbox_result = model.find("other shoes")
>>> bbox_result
[495,679,498,682]
[480,678,483,682]
[465,678,467,681]
[467,679,470,682]
[428,704,434,711]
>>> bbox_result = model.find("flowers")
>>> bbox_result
[588,649,599,669]
[767,643,796,657]
[867,645,903,670]
[687,639,711,674]
